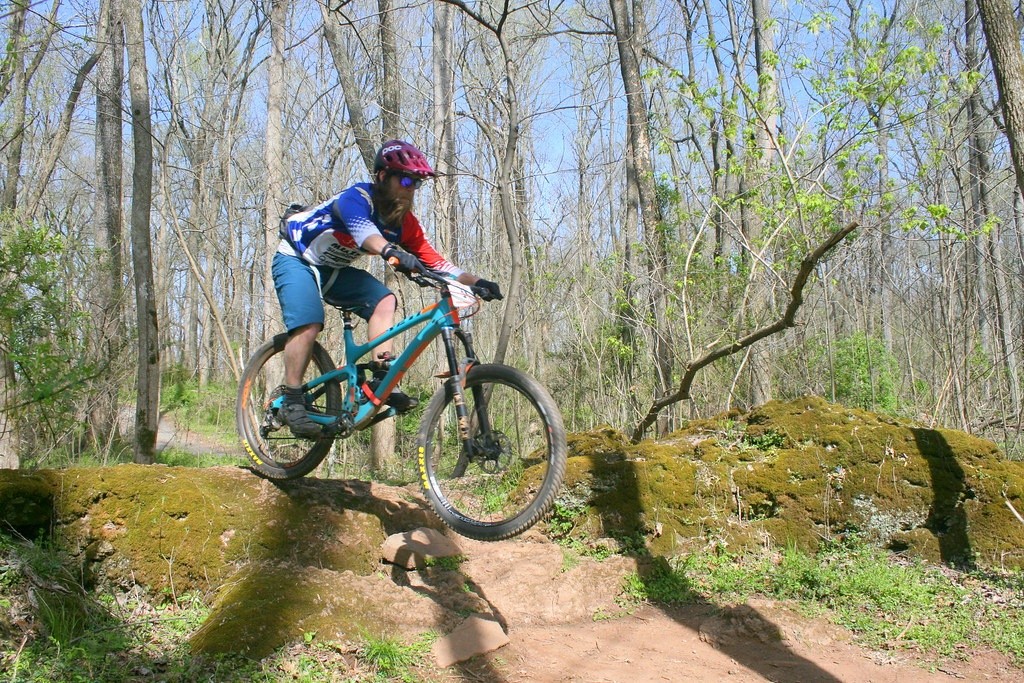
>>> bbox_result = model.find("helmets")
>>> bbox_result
[372,139,435,179]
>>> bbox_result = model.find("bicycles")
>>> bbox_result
[236,256,568,541]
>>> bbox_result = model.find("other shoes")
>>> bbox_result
[366,371,417,411]
[269,386,324,437]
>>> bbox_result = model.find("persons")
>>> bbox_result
[272,140,503,436]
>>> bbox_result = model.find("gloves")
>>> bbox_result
[380,243,431,279]
[470,278,505,303]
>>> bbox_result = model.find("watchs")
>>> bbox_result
[381,243,397,257]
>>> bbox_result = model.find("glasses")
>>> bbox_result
[386,170,424,188]
